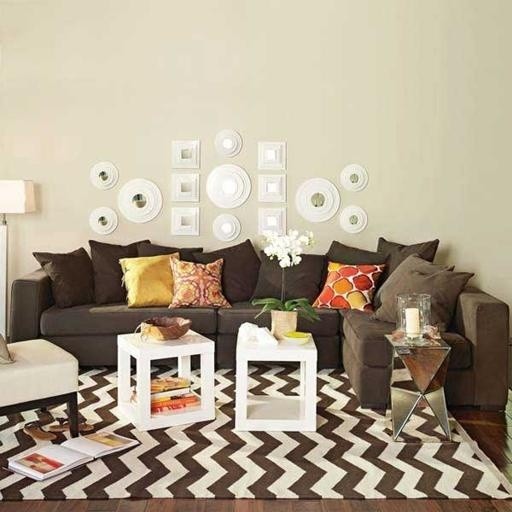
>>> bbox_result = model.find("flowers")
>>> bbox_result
[250,230,321,323]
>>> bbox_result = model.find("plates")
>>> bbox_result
[283,332,312,346]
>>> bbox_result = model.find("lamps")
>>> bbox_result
[1,178,38,341]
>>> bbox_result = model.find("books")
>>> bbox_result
[2,430,143,482]
[130,378,201,417]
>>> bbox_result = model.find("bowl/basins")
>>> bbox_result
[140,316,193,341]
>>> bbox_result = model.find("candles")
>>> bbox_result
[404,308,421,339]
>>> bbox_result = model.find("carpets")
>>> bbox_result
[0,366,511,500]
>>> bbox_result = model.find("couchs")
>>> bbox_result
[8,271,509,409]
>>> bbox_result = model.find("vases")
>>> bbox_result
[266,308,299,339]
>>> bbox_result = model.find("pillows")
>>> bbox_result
[376,235,439,286]
[168,256,232,309]
[371,272,475,331]
[373,254,455,309]
[314,262,386,313]
[193,239,260,303]
[321,240,390,282]
[251,252,325,305]
[120,252,181,309]
[32,246,95,308]
[138,242,203,264]
[88,240,151,306]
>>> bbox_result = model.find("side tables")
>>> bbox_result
[383,334,452,443]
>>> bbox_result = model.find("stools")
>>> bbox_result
[0,338,79,439]
